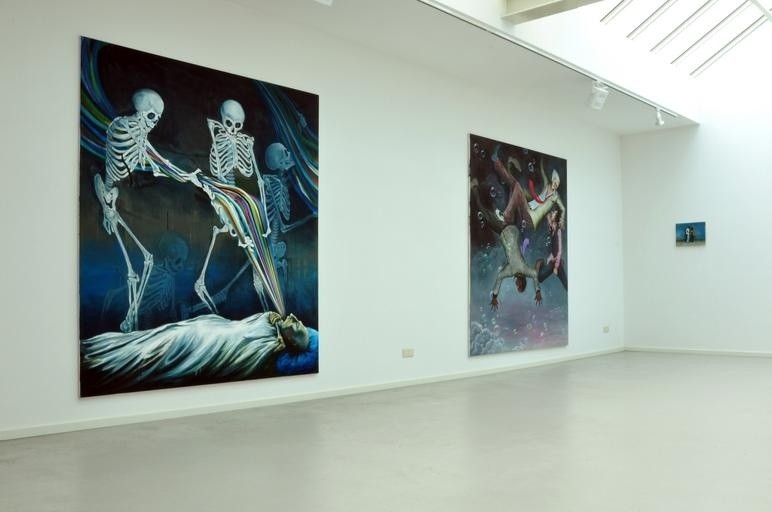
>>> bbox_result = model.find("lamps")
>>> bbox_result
[584,78,609,111]
[653,106,665,129]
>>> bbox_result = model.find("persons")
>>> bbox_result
[470,146,568,310]
